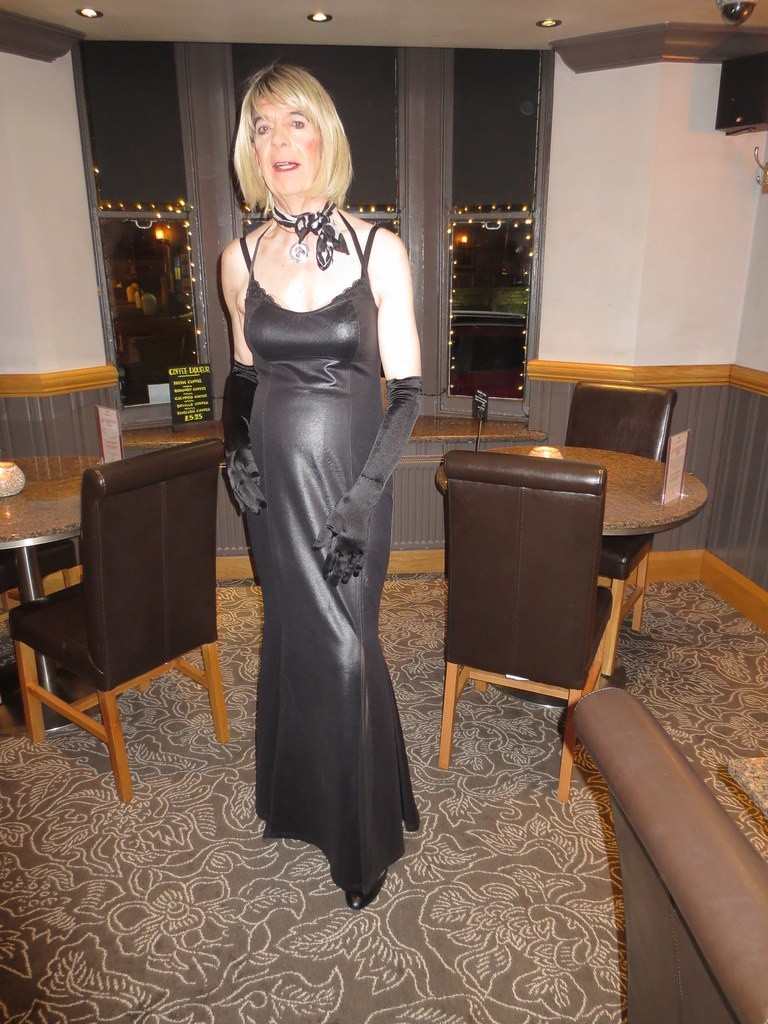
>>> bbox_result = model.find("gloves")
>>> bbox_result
[312,375,423,587]
[221,358,268,517]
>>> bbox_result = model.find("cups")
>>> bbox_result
[527,446,564,460]
[0,462,25,497]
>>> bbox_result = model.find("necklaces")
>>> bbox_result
[277,223,310,262]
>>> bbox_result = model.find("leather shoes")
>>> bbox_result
[344,866,388,910]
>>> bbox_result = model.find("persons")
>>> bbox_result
[220,63,423,909]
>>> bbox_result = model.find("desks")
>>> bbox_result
[0,456,100,740]
[434,446,708,711]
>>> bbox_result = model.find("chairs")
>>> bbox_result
[438,450,614,805]
[564,379,679,680]
[8,437,230,805]
